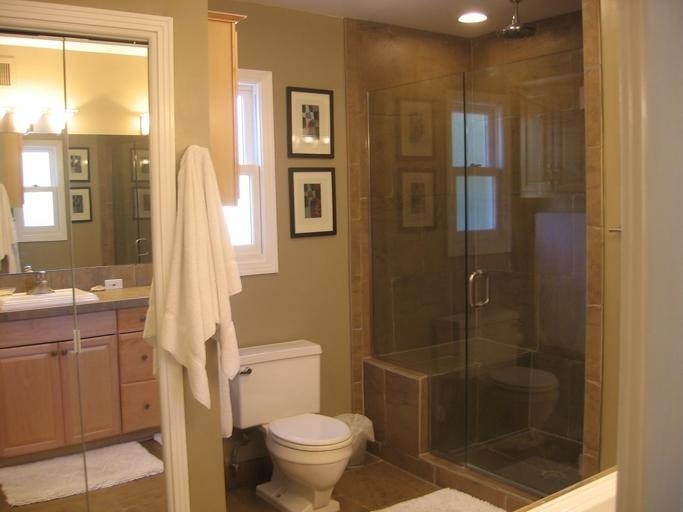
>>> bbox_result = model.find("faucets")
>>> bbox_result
[24,265,34,271]
[27,270,54,294]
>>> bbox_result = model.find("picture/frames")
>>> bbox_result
[129,147,149,221]
[284,86,337,240]
[395,95,438,232]
[68,147,92,224]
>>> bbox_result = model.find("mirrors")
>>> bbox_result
[0,132,151,277]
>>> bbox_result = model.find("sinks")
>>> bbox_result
[0,287,101,313]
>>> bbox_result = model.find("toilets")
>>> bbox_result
[227,338,353,512]
[433,307,560,450]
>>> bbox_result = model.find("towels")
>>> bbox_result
[159,142,246,440]
[0,185,22,273]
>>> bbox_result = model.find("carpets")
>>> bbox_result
[368,486,506,512]
[0,440,164,508]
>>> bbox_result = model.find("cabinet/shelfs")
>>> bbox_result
[518,79,584,200]
[0,309,121,460]
[116,307,159,435]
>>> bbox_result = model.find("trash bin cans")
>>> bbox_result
[334,412,370,467]
